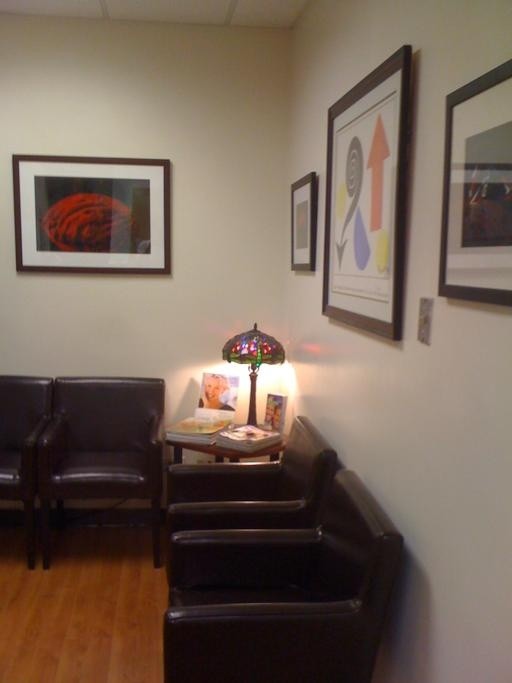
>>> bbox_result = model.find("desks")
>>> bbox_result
[162,413,289,463]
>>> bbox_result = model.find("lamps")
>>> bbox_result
[222,323,288,431]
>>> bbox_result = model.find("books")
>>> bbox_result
[163,416,284,454]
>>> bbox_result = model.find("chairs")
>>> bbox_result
[156,416,342,538]
[33,368,170,570]
[0,371,57,568]
[159,466,410,679]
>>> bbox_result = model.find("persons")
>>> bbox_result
[264,394,279,422]
[198,372,236,411]
[466,160,511,239]
[271,403,283,430]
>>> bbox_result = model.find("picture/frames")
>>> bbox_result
[11,150,172,276]
[435,56,511,307]
[320,40,415,342]
[287,170,320,274]
